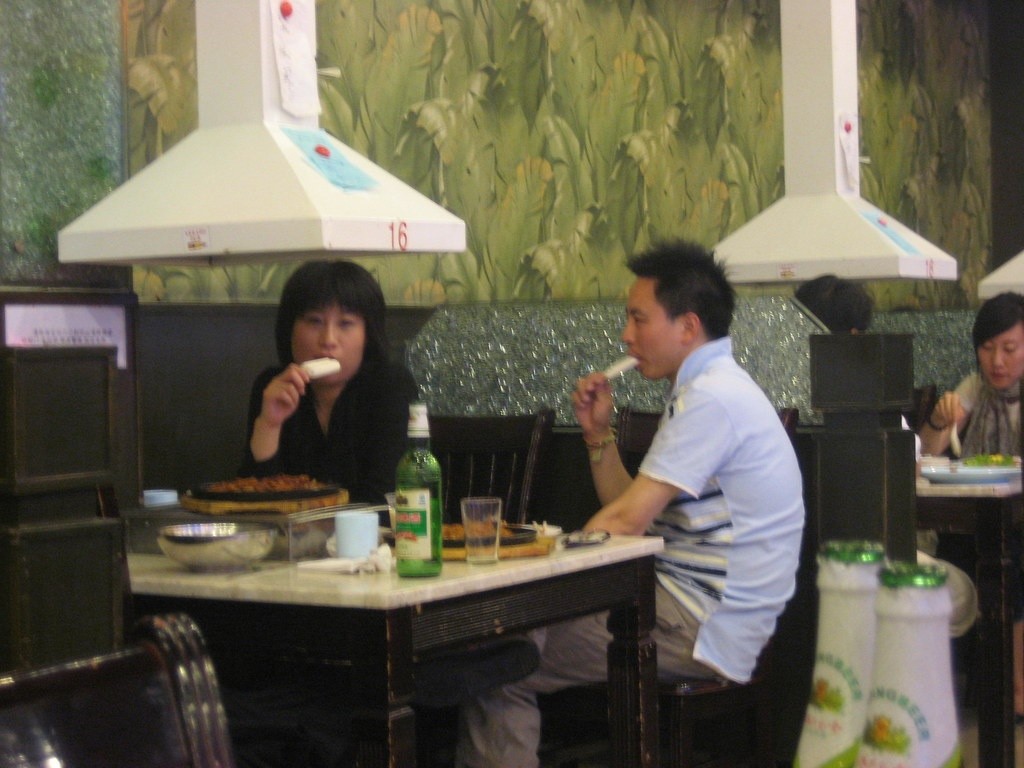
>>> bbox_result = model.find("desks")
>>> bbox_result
[917,454,1024,768]
[128,525,666,768]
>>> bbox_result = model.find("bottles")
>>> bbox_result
[853,565,964,768]
[792,537,885,768]
[395,400,443,578]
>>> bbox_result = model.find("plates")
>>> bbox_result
[443,527,538,545]
[190,481,340,501]
[920,464,1022,486]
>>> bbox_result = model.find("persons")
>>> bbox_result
[797,274,982,676]
[920,292,1023,767]
[243,261,418,528]
[455,241,805,768]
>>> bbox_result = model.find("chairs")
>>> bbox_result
[580,407,799,768]
[413,408,558,663]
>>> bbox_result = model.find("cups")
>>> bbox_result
[460,495,502,565]
[142,489,179,509]
[333,511,380,559]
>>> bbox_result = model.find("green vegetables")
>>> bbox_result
[963,453,1014,468]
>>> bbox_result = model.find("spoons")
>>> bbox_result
[949,422,961,457]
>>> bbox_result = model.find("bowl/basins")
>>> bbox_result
[155,520,279,575]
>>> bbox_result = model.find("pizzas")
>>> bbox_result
[440,522,514,540]
[211,473,326,491]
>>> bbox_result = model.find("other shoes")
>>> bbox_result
[407,629,540,698]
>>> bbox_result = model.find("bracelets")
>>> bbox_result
[583,427,616,462]
[928,416,948,430]
